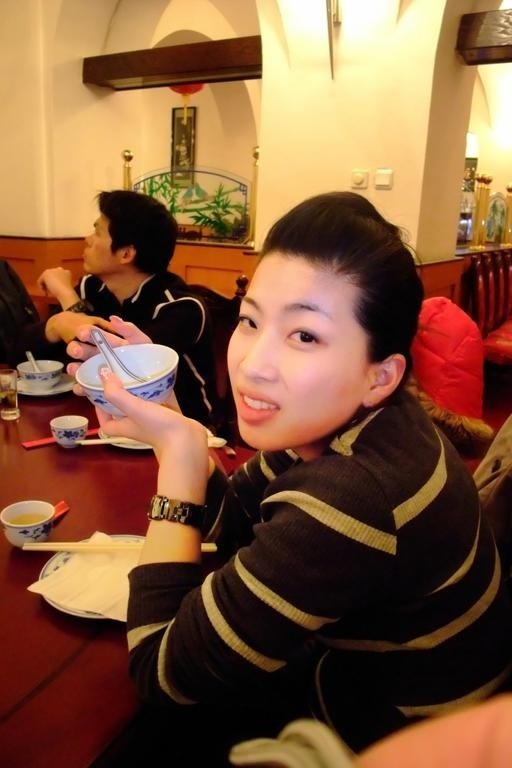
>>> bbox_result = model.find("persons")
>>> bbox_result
[10,189,231,443]
[64,190,511,768]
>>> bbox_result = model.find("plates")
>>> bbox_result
[98,428,153,450]
[17,373,77,397]
[39,534,146,620]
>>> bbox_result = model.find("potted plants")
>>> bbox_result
[188,183,245,241]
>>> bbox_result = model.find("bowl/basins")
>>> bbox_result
[50,415,88,448]
[16,359,64,389]
[76,343,179,419]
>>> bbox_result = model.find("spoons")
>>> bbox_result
[90,328,147,386]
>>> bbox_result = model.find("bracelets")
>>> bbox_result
[66,301,86,313]
[147,494,208,528]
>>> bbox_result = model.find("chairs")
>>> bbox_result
[181,275,249,446]
[470,250,512,415]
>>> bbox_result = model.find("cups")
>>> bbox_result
[0,369,20,421]
[0,500,55,548]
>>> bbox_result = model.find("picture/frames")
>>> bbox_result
[171,106,197,190]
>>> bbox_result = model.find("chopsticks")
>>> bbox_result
[22,541,218,552]
[74,437,131,445]
